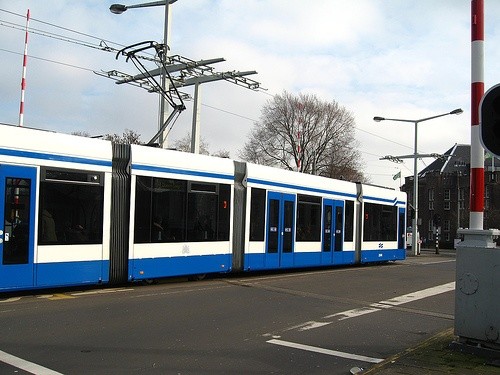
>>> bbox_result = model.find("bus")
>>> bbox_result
[0,40,409,294]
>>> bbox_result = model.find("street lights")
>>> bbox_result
[109,0,177,147]
[373,108,464,256]
